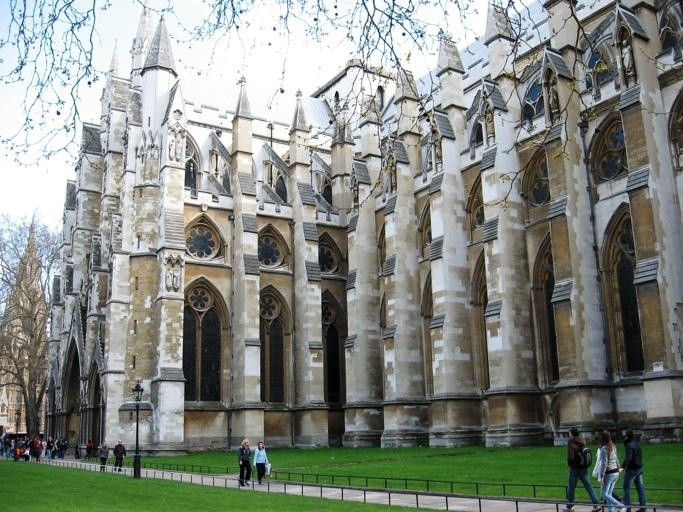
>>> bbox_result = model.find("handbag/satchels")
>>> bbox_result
[265,463,272,477]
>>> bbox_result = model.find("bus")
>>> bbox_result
[0,432,44,442]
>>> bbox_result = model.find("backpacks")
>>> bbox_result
[578,448,592,467]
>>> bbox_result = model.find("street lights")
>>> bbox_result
[13,407,21,461]
[129,378,144,477]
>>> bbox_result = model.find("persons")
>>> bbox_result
[561,429,601,512]
[0,434,68,463]
[74,441,80,460]
[84,440,93,459]
[253,441,268,485]
[99,441,108,472]
[596,430,625,512]
[591,437,623,504]
[621,430,647,512]
[114,440,126,471]
[238,439,252,486]
